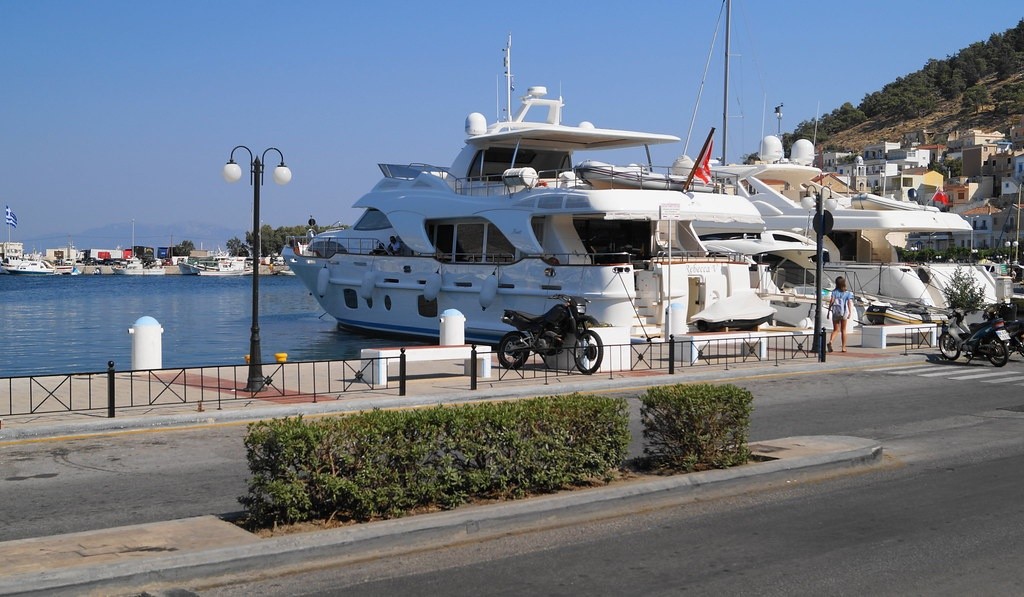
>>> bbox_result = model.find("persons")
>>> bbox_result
[387,236,401,255]
[1002,258,1017,281]
[827,276,853,352]
[369,243,388,255]
[289,236,300,255]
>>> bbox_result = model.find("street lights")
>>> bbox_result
[223,146,292,392]
[1004,240,1019,279]
[800,184,838,353]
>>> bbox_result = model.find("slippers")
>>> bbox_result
[827,343,833,352]
[842,348,847,352]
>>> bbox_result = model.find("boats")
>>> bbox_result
[279,269,295,275]
[283,0,1003,350]
[1,255,60,276]
[178,249,247,275]
[198,269,253,275]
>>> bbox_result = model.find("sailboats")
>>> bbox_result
[111,219,167,275]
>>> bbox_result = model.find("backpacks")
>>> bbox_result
[832,290,848,321]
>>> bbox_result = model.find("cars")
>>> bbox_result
[85,257,98,266]
[105,258,122,266]
[76,257,82,263]
[162,258,173,266]
[64,258,75,266]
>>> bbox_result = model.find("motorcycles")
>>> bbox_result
[938,308,1011,367]
[983,303,1024,358]
[497,294,604,376]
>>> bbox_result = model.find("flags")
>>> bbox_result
[6,205,17,228]
[694,139,714,185]
[934,190,948,204]
